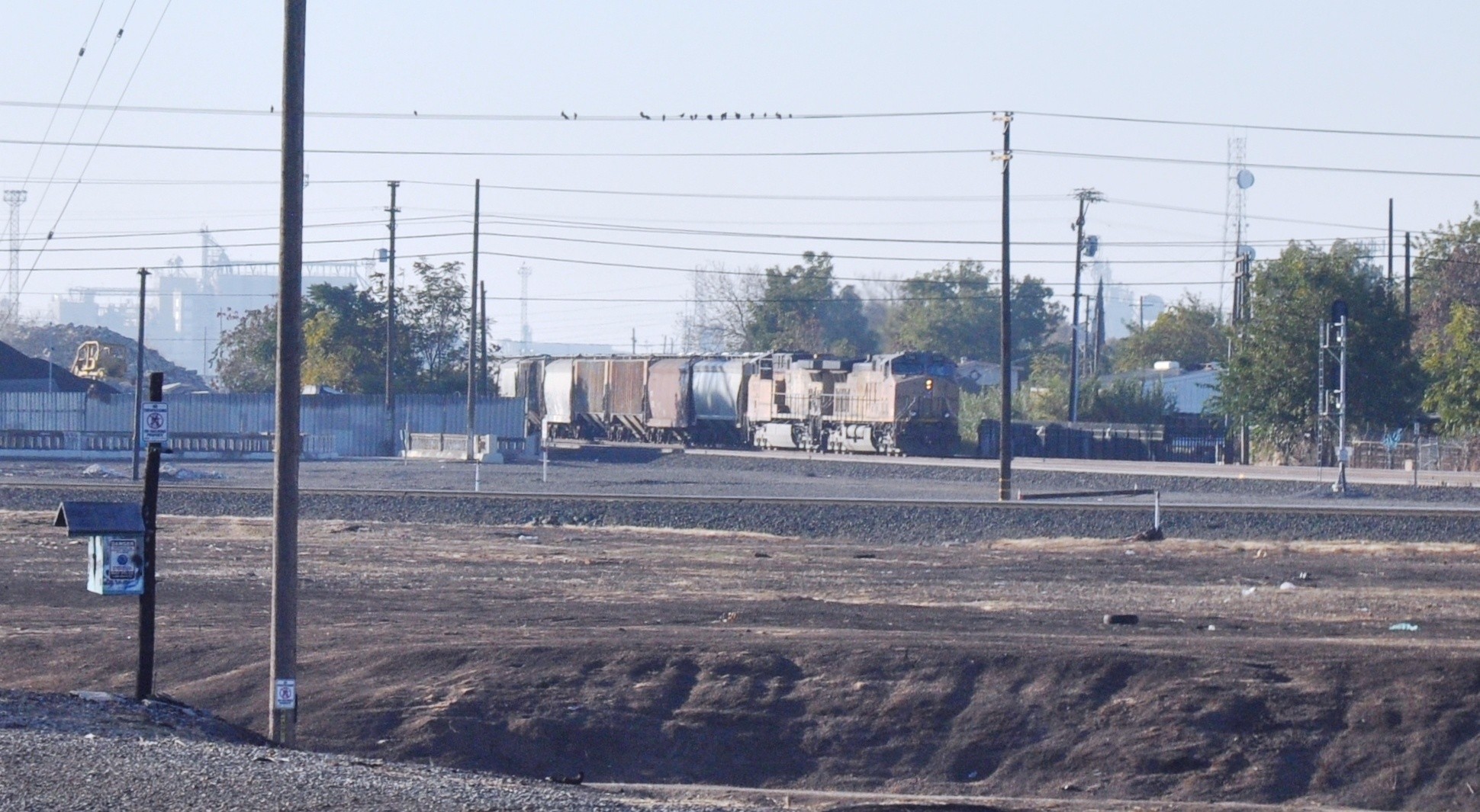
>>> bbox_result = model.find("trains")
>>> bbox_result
[447,347,965,459]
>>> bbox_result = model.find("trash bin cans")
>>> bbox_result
[52,500,145,595]
